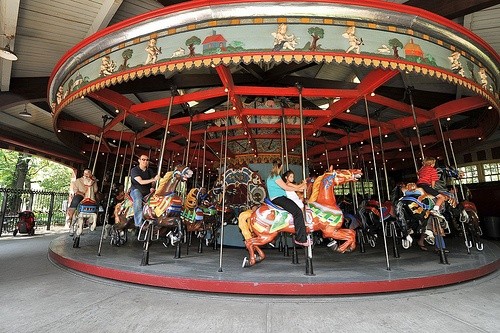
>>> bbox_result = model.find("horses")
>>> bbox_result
[238,158,482,269]
[68,164,237,240]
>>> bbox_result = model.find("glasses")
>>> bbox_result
[142,158,149,161]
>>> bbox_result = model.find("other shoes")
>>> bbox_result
[295,239,309,246]
[431,209,445,218]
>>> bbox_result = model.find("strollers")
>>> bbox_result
[13,211,35,236]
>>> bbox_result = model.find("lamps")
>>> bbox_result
[0,34,18,61]
[19,104,32,117]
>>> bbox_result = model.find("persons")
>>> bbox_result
[67,168,99,223]
[391,179,405,205]
[416,158,446,218]
[444,184,458,239]
[342,190,392,204]
[128,154,161,230]
[96,180,125,226]
[281,169,307,222]
[266,158,312,246]
